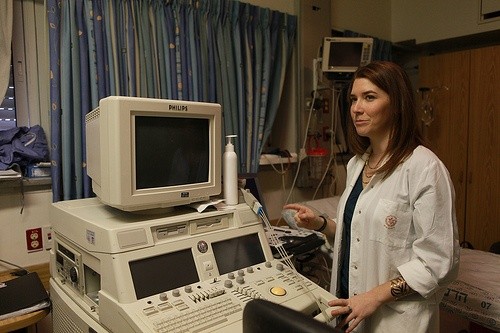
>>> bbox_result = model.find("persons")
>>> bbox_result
[283,62,460,333]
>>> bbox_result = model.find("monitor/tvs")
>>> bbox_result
[85,96,222,215]
[322,37,373,72]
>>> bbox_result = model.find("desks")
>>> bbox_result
[0,270,50,333]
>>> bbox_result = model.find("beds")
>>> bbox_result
[283,195,500,333]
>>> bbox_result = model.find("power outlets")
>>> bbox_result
[26,227,43,253]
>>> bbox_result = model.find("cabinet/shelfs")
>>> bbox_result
[418,45,500,254]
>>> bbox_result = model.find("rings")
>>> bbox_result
[347,305,352,313]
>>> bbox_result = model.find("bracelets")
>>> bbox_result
[313,216,327,232]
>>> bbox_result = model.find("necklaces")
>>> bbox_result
[362,157,380,184]
